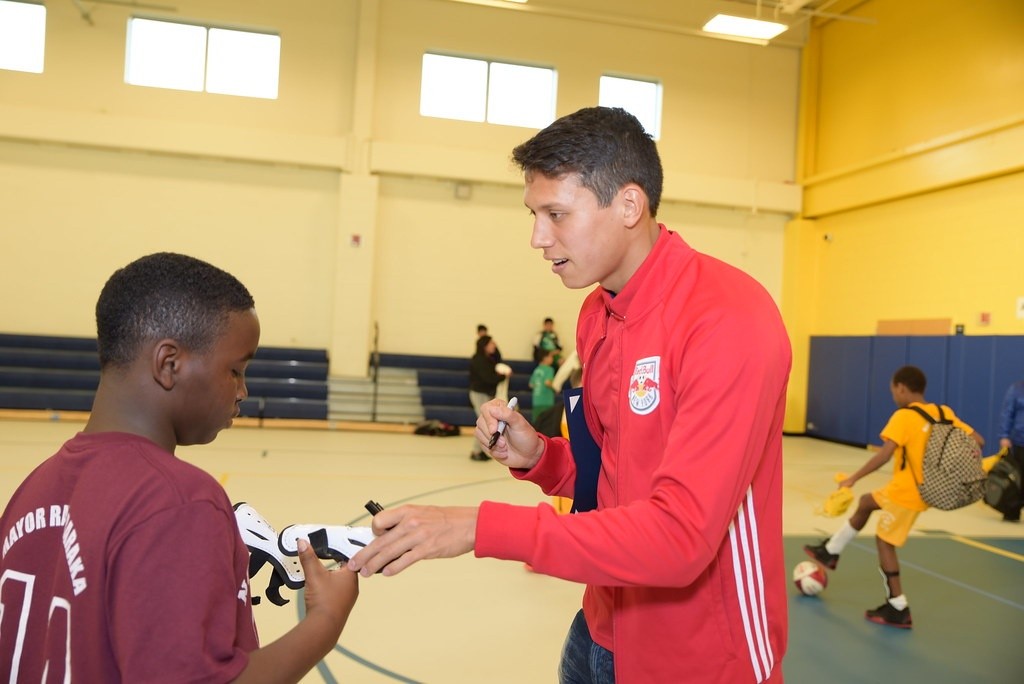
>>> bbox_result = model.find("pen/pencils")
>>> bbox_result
[487,396,519,450]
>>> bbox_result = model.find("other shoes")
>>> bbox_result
[471,450,492,461]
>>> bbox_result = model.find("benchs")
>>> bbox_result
[1,333,573,428]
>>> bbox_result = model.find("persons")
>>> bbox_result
[999,378,1024,521]
[801,366,985,629]
[468,318,584,515]
[348,107,789,684]
[0,251,359,683]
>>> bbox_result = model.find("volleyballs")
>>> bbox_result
[792,561,828,596]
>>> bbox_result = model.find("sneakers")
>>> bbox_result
[865,600,912,628]
[803,538,839,570]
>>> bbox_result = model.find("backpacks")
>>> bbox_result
[904,403,988,511]
[983,456,1023,515]
